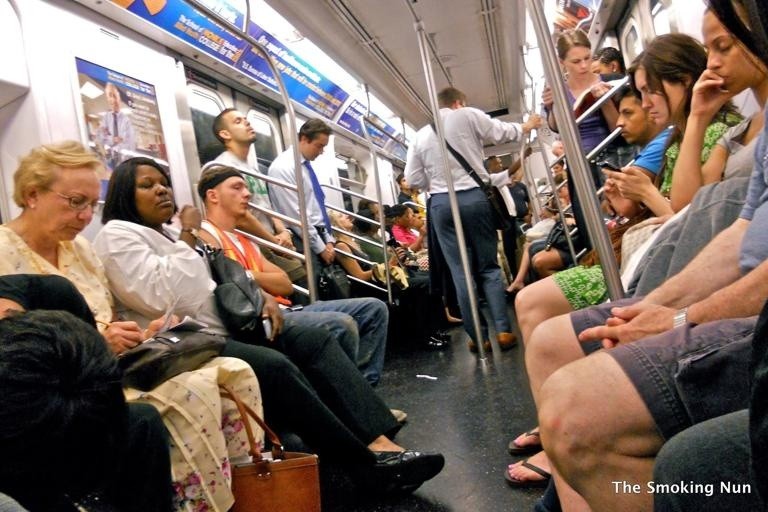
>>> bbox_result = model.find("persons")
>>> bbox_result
[404,88,545,353]
[378,173,433,282]
[353,211,448,348]
[1,275,174,511]
[92,156,445,499]
[197,165,407,422]
[85,80,135,149]
[507,175,535,274]
[202,108,314,306]
[548,29,630,250]
[268,119,334,306]
[502,35,746,486]
[0,140,264,510]
[506,0,768,510]
[654,293,767,510]
[484,147,534,286]
[507,150,584,292]
[523,108,768,508]
[326,210,407,368]
[593,48,624,74]
[611,85,680,183]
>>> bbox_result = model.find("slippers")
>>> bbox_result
[505,459,553,488]
[509,426,543,454]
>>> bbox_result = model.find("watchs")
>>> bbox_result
[674,308,686,326]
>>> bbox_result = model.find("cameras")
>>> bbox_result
[387,239,407,263]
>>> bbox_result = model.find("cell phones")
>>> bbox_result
[547,205,560,219]
[260,317,273,340]
[598,161,623,172]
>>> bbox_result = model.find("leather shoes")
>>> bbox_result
[497,332,518,351]
[421,335,447,351]
[390,408,408,424]
[429,335,449,347]
[374,449,446,481]
[358,469,426,504]
[464,339,492,353]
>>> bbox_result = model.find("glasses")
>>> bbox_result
[37,183,105,214]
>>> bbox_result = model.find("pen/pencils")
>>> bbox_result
[96,319,110,326]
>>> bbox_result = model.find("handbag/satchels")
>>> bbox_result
[120,326,232,392]
[545,219,580,256]
[218,385,322,512]
[209,246,270,342]
[429,120,518,233]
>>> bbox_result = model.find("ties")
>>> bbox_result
[304,161,332,236]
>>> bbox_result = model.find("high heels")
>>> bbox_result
[504,283,524,295]
